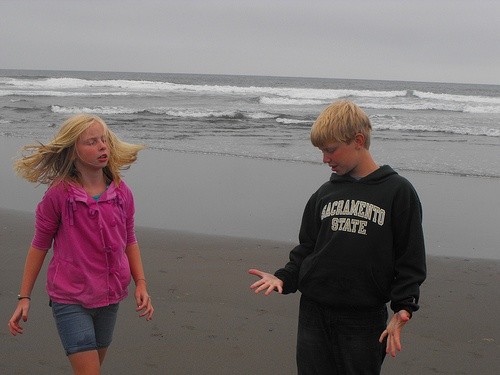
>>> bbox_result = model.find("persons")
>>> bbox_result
[236,98,426,375]
[5,113,154,375]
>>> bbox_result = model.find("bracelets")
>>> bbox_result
[132,277,149,284]
[14,294,30,299]
